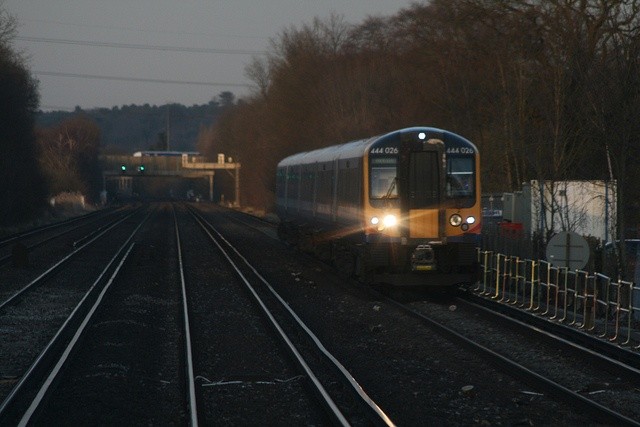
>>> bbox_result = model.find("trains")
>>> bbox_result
[274,126,482,286]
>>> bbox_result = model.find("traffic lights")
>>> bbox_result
[138,162,145,174]
[121,162,126,173]
